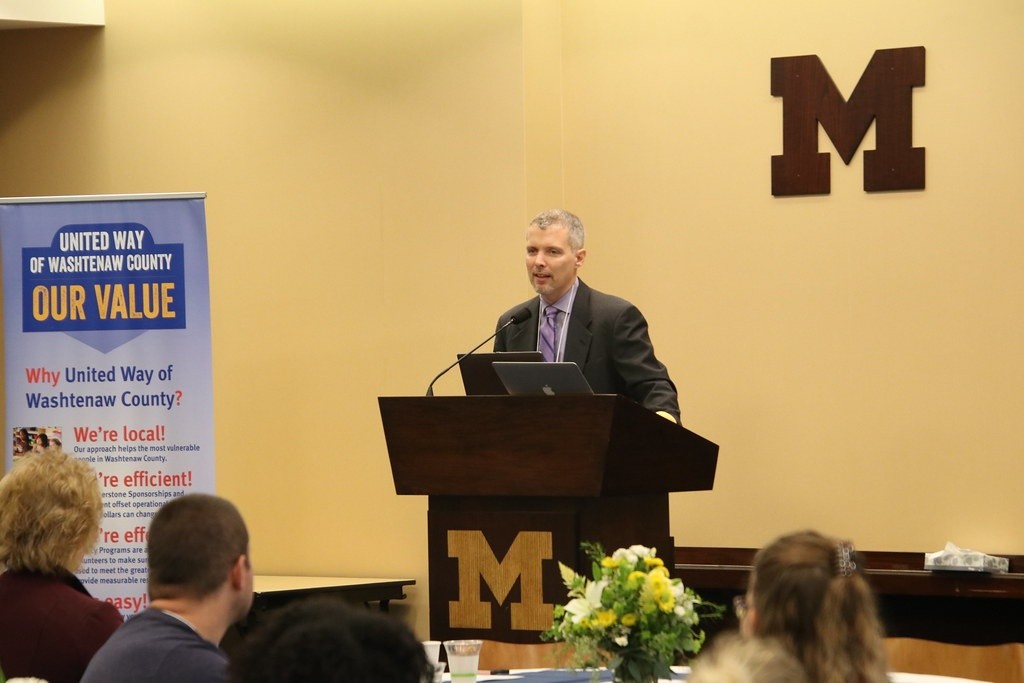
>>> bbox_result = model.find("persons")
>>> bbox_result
[492,206,686,426]
[14,428,61,455]
[79,493,252,683]
[686,529,890,683]
[222,597,435,683]
[0,449,122,682]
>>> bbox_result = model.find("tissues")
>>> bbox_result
[924,540,1009,574]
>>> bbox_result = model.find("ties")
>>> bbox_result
[540,306,559,362]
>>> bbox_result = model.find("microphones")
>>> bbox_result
[425,307,533,397]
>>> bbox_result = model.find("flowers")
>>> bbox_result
[539,540,705,683]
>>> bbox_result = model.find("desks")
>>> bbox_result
[251,576,417,611]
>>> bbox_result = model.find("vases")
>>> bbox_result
[615,661,658,683]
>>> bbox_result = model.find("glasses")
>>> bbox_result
[733,594,752,619]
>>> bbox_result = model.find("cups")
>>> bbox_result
[444,640,484,683]
[419,640,446,683]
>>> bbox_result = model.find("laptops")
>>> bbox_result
[456,351,594,395]
